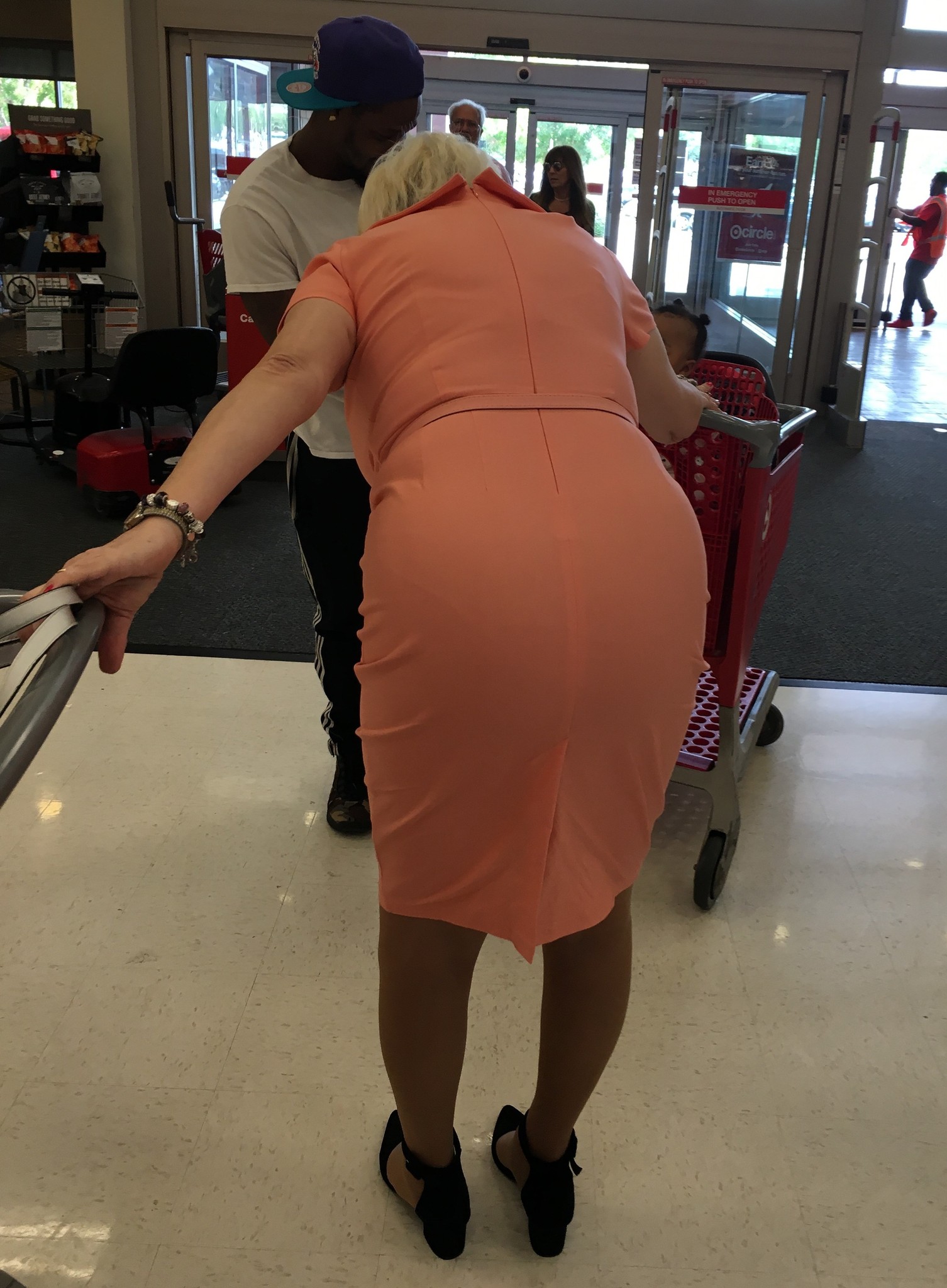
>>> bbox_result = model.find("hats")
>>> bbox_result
[275,14,426,111]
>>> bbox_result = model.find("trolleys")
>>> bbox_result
[638,351,818,913]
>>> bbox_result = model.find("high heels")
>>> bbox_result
[379,1109,471,1261]
[492,1103,584,1258]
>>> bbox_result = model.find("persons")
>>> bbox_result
[447,99,509,182]
[652,299,712,385]
[217,14,425,833]
[16,133,715,1258]
[528,145,595,236]
[886,171,947,328]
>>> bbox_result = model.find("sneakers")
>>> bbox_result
[922,309,938,327]
[326,757,372,835]
[886,309,914,328]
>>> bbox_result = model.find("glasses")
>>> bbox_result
[450,118,482,129]
[543,162,567,171]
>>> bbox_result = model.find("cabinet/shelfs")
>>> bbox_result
[0,134,107,392]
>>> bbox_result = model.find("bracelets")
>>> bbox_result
[119,493,206,568]
